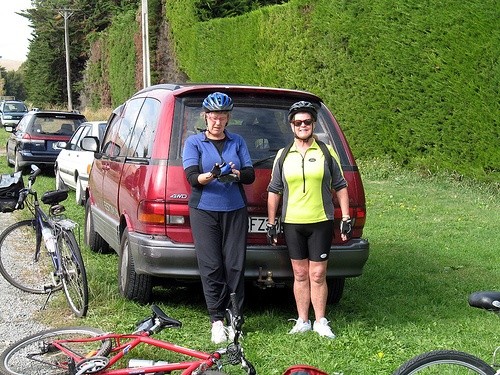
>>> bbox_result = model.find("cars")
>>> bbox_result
[53,120,109,208]
[0,101,29,132]
[5,107,89,177]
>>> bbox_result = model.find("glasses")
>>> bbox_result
[291,120,313,126]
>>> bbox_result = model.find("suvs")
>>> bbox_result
[80,82,371,306]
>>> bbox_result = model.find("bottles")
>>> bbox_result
[41,227,55,252]
[128,359,168,367]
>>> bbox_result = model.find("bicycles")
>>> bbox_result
[389,291,500,375]
[0,164,90,320]
[0,292,258,375]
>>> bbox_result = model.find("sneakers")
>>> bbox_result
[211,320,228,344]
[228,325,244,342]
[313,317,336,339]
[286,318,312,335]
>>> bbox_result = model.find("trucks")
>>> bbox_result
[0,96,16,112]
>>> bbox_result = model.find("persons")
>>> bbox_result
[183,92,256,344]
[267,101,351,339]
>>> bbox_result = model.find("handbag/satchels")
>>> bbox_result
[1,170,26,213]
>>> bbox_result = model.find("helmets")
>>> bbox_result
[203,92,234,113]
[288,101,318,121]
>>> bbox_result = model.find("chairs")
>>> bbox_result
[5,106,10,111]
[33,124,45,133]
[61,124,73,132]
